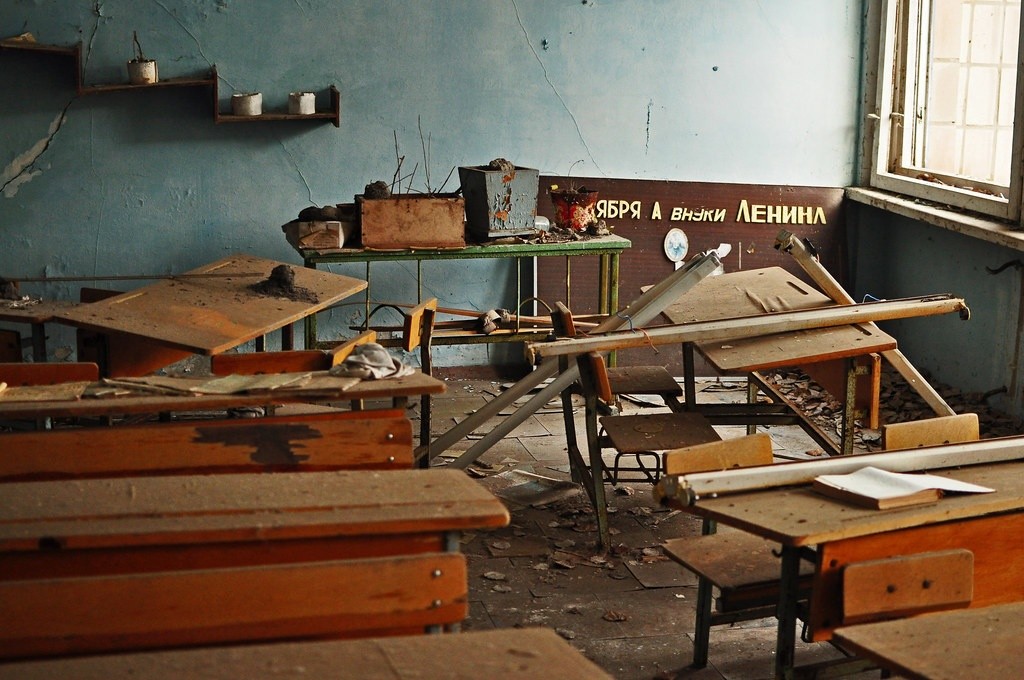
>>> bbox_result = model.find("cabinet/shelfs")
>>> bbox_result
[0,36,343,129]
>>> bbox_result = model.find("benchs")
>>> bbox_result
[2,413,420,485]
[259,298,441,479]
[693,451,1024,680]
[1,551,484,666]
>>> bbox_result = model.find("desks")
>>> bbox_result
[2,467,512,640]
[51,254,374,380]
[285,228,632,390]
[834,601,1023,680]
[0,628,620,680]
[640,266,897,459]
[0,366,448,480]
[0,294,82,365]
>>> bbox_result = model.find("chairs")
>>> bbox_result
[841,550,983,658]
[568,331,732,556]
[659,431,848,669]
[0,359,100,388]
[210,349,334,375]
[883,412,982,453]
[550,300,685,493]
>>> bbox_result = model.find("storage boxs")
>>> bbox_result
[282,218,354,249]
[355,194,466,251]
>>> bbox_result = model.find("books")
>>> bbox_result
[809,466,999,510]
